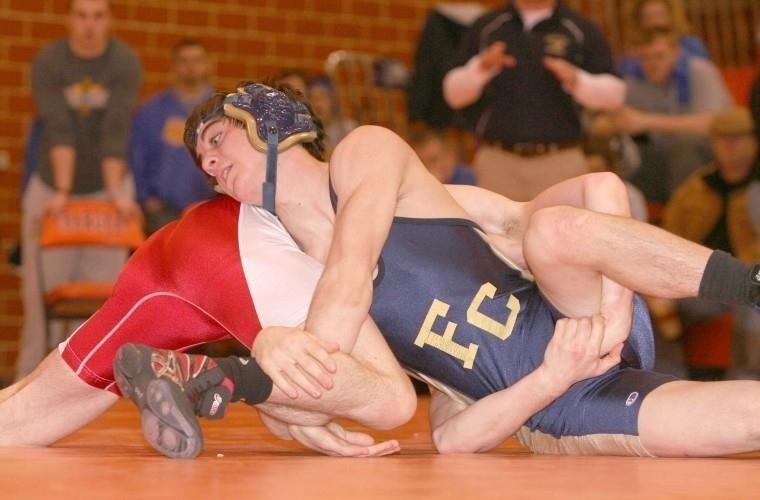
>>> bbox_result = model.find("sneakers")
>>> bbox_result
[112,341,235,460]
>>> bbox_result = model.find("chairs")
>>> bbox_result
[37,202,148,366]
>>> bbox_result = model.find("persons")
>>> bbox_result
[2,81,759,458]
[403,1,759,381]
[128,36,219,239]
[330,49,473,183]
[6,0,141,270]
[271,67,361,164]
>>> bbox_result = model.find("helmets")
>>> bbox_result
[212,82,317,195]
